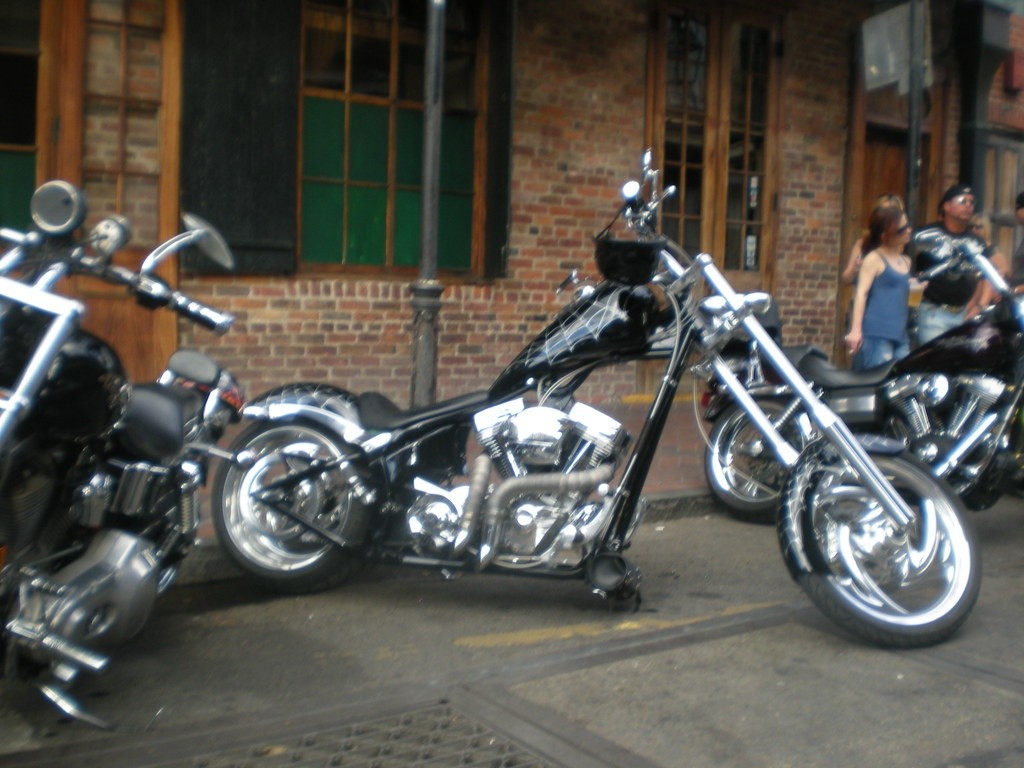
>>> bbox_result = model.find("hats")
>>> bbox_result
[1015,192,1024,209]
[937,183,975,207]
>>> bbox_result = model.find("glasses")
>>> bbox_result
[897,220,914,233]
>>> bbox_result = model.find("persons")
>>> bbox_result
[842,194,914,373]
[907,183,1024,348]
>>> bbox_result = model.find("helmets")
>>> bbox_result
[591,235,665,285]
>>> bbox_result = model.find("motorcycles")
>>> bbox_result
[1,170,254,733]
[208,143,986,652]
[687,277,1023,529]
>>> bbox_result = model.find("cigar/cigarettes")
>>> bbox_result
[849,349,854,354]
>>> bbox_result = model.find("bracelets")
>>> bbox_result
[976,303,982,310]
